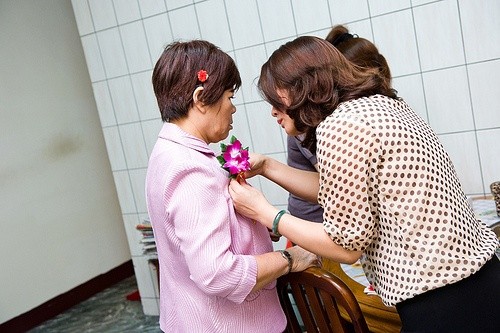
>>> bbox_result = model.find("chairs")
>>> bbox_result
[276,266,369,333]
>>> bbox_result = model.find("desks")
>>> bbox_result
[321,258,400,333]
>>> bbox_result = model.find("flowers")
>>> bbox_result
[216,135,252,178]
[197,70,208,82]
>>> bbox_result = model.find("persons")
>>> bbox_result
[287,25,392,247]
[228,36,500,333]
[145,40,322,333]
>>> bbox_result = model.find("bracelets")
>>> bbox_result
[273,210,290,237]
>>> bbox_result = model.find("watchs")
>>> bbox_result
[275,250,294,274]
